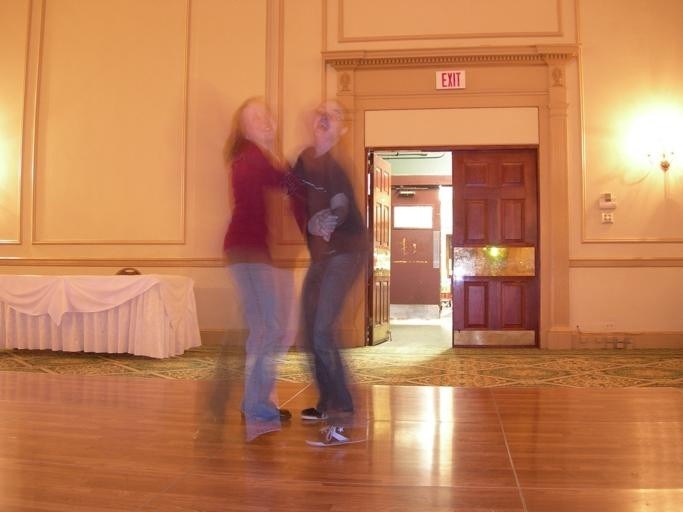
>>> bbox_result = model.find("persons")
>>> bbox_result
[221,96,307,444]
[288,97,373,447]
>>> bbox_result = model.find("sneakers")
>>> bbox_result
[276,407,290,417]
[300,407,354,418]
[305,422,369,446]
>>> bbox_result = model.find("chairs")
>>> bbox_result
[114,267,143,276]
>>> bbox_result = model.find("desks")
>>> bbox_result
[0,274,202,359]
